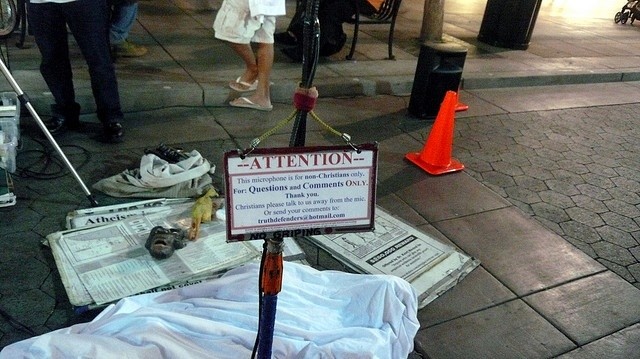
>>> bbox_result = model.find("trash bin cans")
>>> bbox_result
[406,39,469,119]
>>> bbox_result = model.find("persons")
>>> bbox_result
[342,237,359,248]
[354,232,372,243]
[26,0,124,144]
[372,230,384,237]
[375,220,395,231]
[213,0,276,112]
[106,1,150,65]
[273,0,385,62]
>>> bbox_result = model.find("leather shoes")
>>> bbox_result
[282,48,303,63]
[102,122,125,144]
[41,105,80,136]
[273,32,298,47]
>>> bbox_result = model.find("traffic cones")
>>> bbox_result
[403,90,465,176]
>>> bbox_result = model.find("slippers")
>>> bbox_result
[230,97,273,111]
[229,77,272,92]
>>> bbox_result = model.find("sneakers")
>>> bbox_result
[112,40,147,57]
